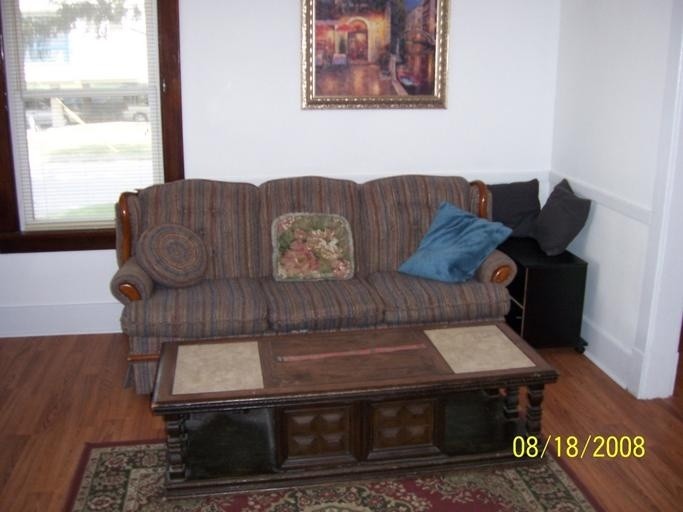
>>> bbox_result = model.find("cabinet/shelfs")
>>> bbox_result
[496,236,590,353]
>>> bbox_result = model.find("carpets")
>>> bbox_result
[61,432,609,512]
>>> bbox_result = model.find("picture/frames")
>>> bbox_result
[300,0,450,110]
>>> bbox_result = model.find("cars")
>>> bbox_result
[120,98,150,122]
[24,97,67,127]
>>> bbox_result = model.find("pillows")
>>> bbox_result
[136,223,208,286]
[524,178,592,256]
[396,201,513,284]
[271,212,355,283]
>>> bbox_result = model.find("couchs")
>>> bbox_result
[109,176,518,396]
